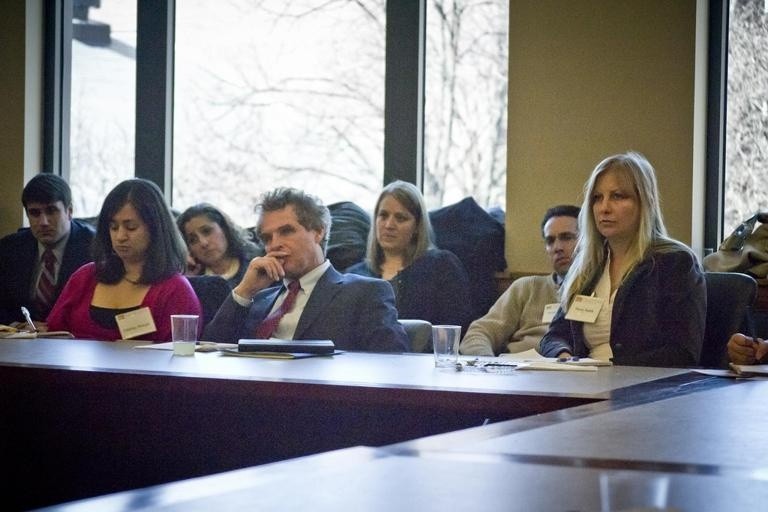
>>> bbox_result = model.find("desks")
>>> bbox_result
[41,373,766,512]
[2,335,697,503]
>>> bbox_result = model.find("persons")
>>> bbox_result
[539,153,708,368]
[459,205,580,356]
[177,203,263,290]
[718,331,768,368]
[340,180,476,352]
[0,173,97,332]
[26,179,203,342]
[202,187,411,353]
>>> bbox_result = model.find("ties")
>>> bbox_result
[32,249,58,329]
[256,280,299,339]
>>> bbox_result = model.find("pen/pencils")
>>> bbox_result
[557,355,581,362]
[196,341,216,344]
[746,306,761,365]
[21,305,36,331]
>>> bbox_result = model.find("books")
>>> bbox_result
[238,339,335,354]
[219,346,346,359]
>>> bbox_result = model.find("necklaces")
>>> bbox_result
[122,275,139,284]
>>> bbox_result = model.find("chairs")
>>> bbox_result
[184,267,760,370]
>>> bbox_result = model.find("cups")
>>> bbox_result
[170,313,199,355]
[433,324,462,367]
